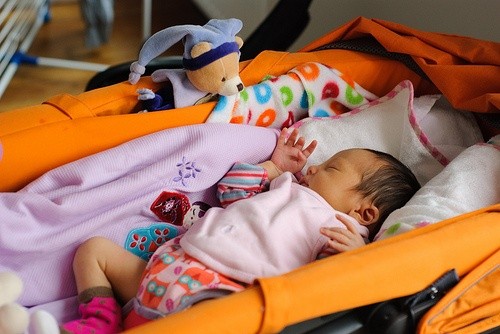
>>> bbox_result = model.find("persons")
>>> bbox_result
[58,125,422,334]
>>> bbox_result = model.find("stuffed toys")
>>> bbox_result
[128,16,243,115]
